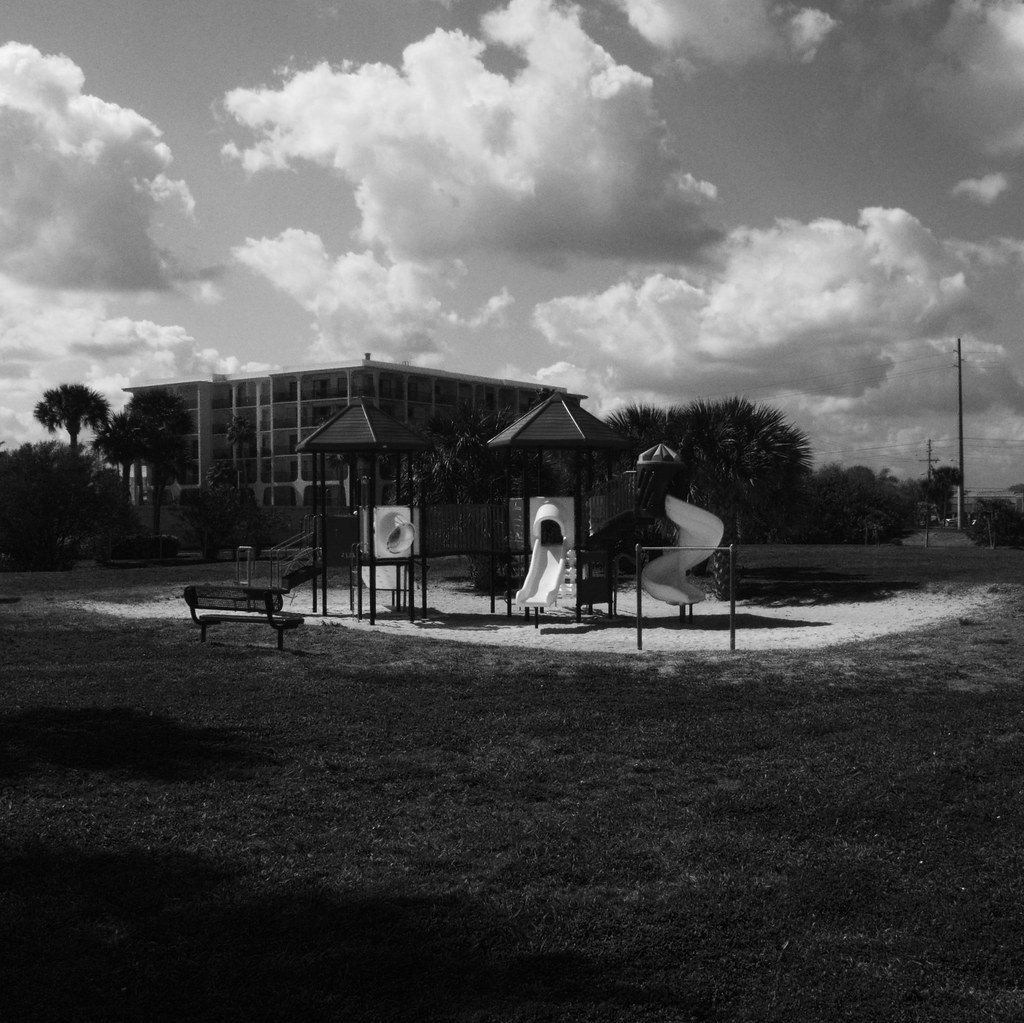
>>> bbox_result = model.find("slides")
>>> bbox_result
[515,537,567,607]
[640,495,725,605]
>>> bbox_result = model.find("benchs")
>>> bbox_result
[184,585,306,650]
